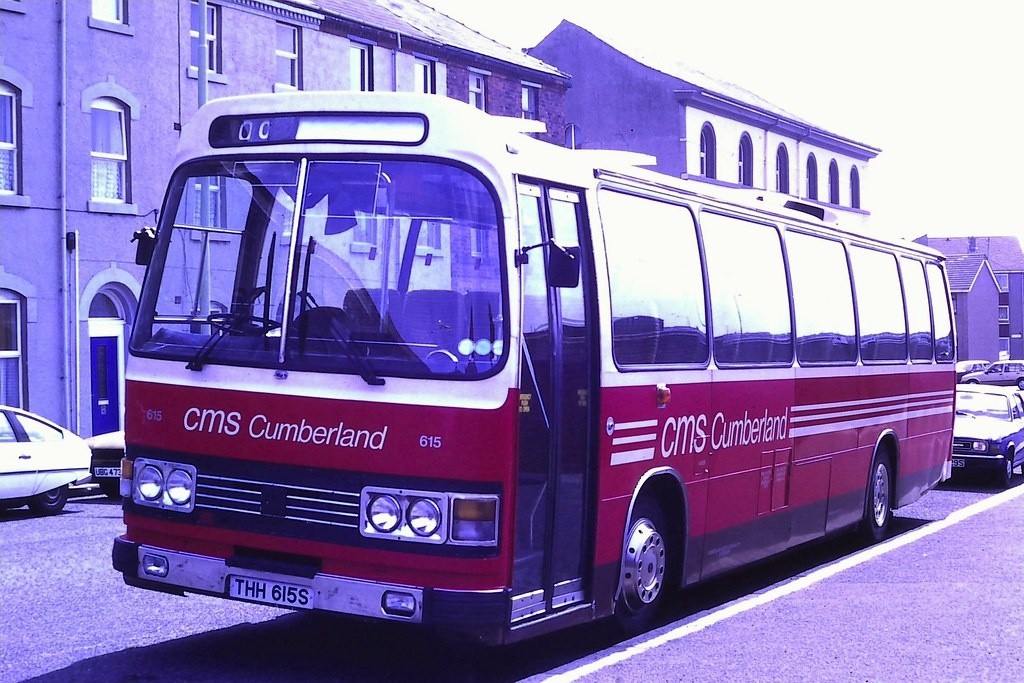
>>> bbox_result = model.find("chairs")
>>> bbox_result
[291,289,879,365]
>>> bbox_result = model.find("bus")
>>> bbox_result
[112,91,957,653]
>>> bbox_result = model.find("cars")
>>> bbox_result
[0,404,125,518]
[951,359,1024,486]
